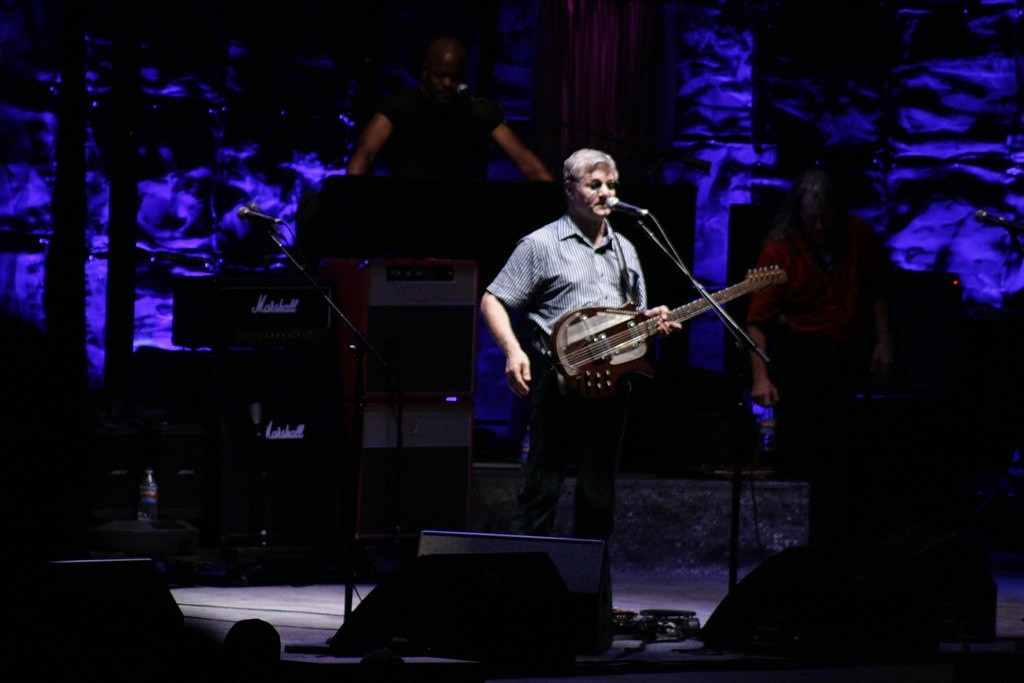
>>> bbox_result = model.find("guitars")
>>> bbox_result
[550,263,789,397]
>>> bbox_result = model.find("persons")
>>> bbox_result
[745,169,894,474]
[480,147,683,545]
[344,35,554,181]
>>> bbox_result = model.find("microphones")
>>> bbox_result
[236,207,287,226]
[605,197,649,218]
[974,210,1024,233]
[456,84,477,104]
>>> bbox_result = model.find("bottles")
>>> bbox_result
[136,470,158,521]
[520,425,530,462]
[759,402,777,449]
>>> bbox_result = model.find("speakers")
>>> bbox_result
[323,259,480,404]
[196,348,352,541]
[41,559,184,627]
[699,530,998,658]
[329,531,616,659]
[356,403,474,541]
